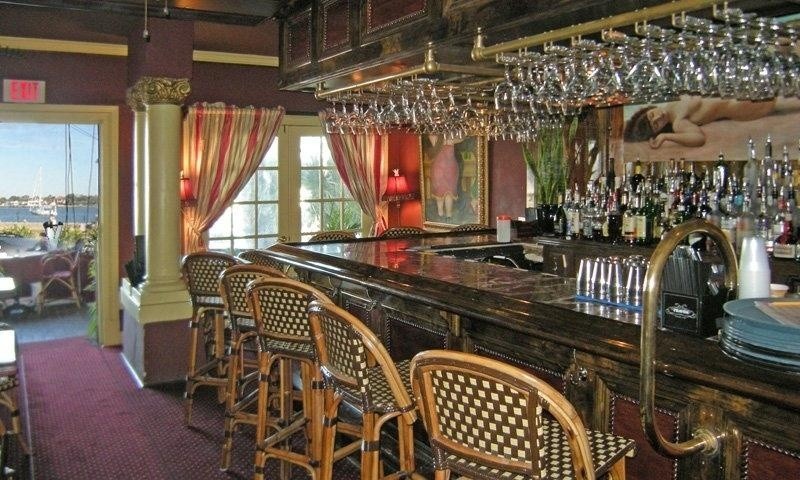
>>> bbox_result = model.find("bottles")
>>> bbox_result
[553,135,800,262]
[496,215,511,243]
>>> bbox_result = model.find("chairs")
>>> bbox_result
[37,238,84,317]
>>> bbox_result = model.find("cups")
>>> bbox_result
[771,284,790,298]
[737,237,771,299]
[575,254,650,307]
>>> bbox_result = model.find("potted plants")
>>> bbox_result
[521,116,579,234]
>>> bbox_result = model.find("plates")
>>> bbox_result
[719,295,799,374]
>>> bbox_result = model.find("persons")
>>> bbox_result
[624,91,799,149]
[427,133,467,219]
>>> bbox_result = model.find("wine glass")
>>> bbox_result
[325,78,565,143]
[494,9,800,115]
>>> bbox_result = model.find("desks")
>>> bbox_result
[0,328,39,456]
[0,250,47,315]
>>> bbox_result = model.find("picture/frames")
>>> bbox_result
[419,133,489,232]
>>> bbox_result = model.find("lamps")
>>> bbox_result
[382,169,419,227]
[180,170,198,211]
